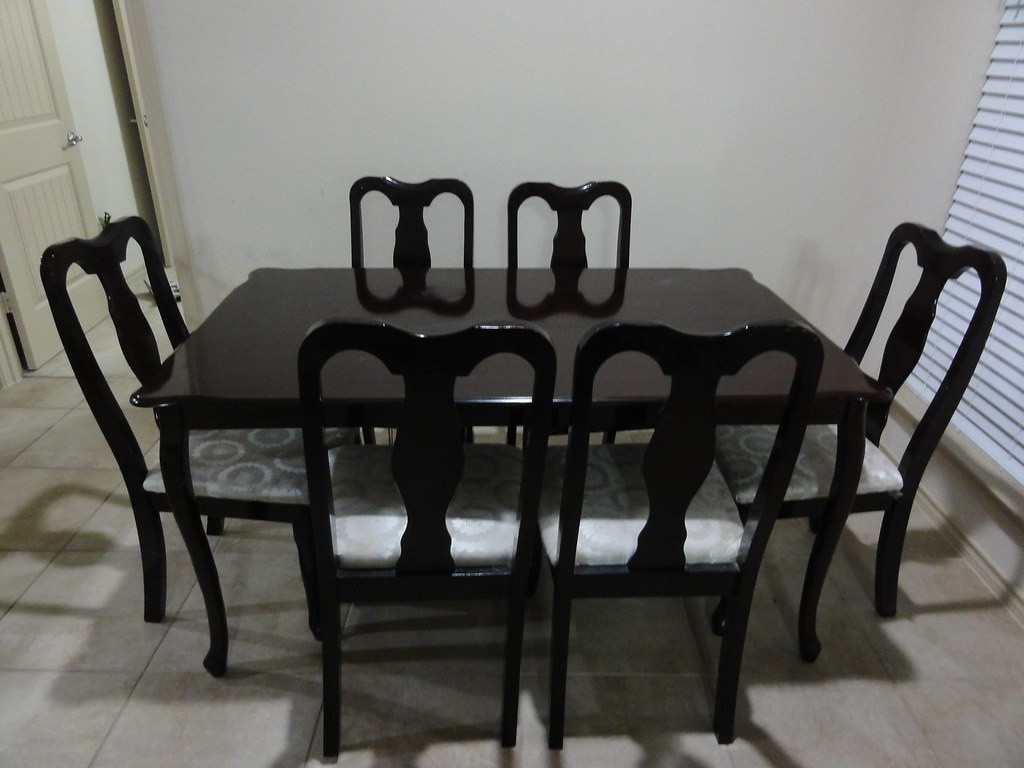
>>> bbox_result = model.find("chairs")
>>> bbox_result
[532,316,824,746]
[39,214,345,642]
[505,179,634,451]
[299,316,555,762]
[710,223,1006,633]
[347,173,478,446]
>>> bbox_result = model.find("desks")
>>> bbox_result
[128,267,892,663]
